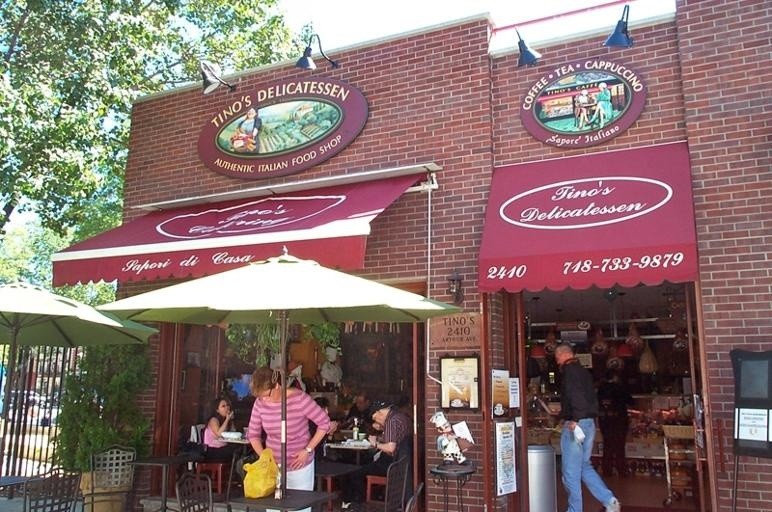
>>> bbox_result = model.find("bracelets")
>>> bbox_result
[304,446,313,455]
[231,418,235,421]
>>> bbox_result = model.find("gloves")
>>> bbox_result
[574,425,586,444]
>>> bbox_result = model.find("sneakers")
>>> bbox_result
[606,498,621,512]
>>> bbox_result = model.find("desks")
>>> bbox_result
[222,436,251,500]
[326,444,380,464]
[0,476,29,491]
[225,488,334,512]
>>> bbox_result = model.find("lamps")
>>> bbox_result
[295,34,341,73]
[602,1,634,52]
[195,57,239,95]
[514,29,543,69]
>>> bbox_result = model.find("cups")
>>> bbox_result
[359,433,367,440]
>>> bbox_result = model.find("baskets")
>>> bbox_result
[526,429,553,445]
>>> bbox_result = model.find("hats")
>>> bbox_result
[368,399,390,418]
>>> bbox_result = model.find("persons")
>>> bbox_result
[313,374,412,438]
[574,88,595,130]
[238,107,262,154]
[554,344,622,512]
[246,366,332,512]
[589,82,614,128]
[307,396,356,510]
[598,364,637,478]
[347,399,412,503]
[202,396,237,462]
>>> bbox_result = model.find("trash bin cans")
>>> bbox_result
[528,445,557,512]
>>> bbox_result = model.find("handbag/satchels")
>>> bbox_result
[180,441,204,460]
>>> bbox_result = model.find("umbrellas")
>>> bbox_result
[1,284,160,473]
[95,246,467,505]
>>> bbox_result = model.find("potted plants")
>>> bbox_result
[52,343,153,511]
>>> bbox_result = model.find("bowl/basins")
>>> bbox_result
[222,431,243,439]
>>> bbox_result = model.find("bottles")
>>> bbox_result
[352,419,359,441]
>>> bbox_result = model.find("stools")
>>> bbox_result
[315,464,361,510]
[366,474,387,510]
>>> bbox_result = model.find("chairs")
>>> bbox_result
[89,446,135,511]
[190,424,228,495]
[26,467,81,512]
[343,457,409,512]
[174,471,213,512]
